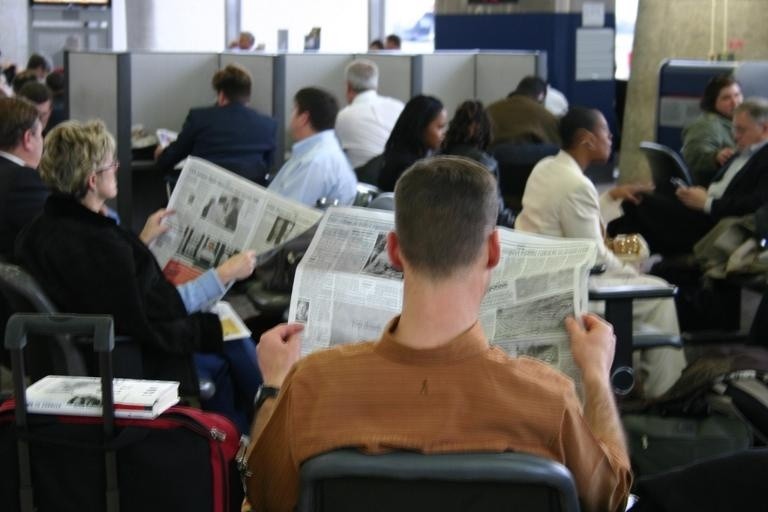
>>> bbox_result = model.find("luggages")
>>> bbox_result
[0,315,244,511]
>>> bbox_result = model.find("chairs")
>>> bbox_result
[0,146,768,512]
